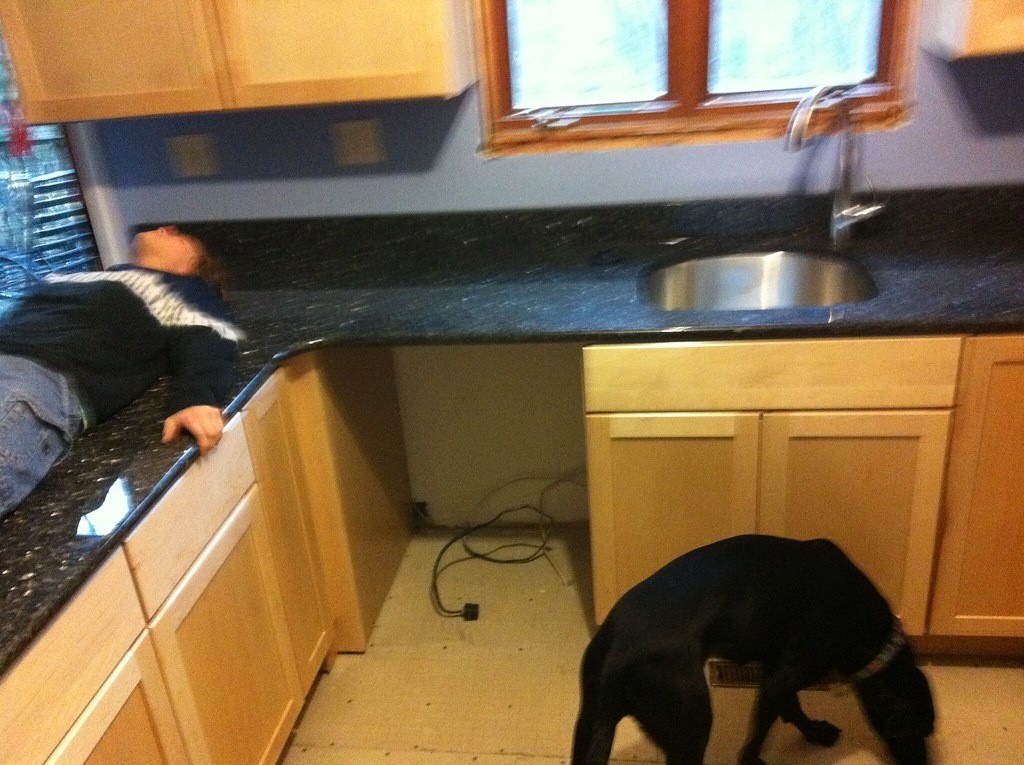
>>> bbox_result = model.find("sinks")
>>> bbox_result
[645,248,880,311]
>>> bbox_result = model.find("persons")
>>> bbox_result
[0,226,237,515]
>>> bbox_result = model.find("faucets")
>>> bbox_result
[783,79,893,247]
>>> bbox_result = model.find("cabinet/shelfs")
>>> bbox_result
[581,331,965,661]
[0,0,476,121]
[927,336,1024,666]
[0,342,412,765]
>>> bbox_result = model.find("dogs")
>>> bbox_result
[571,535,934,765]
